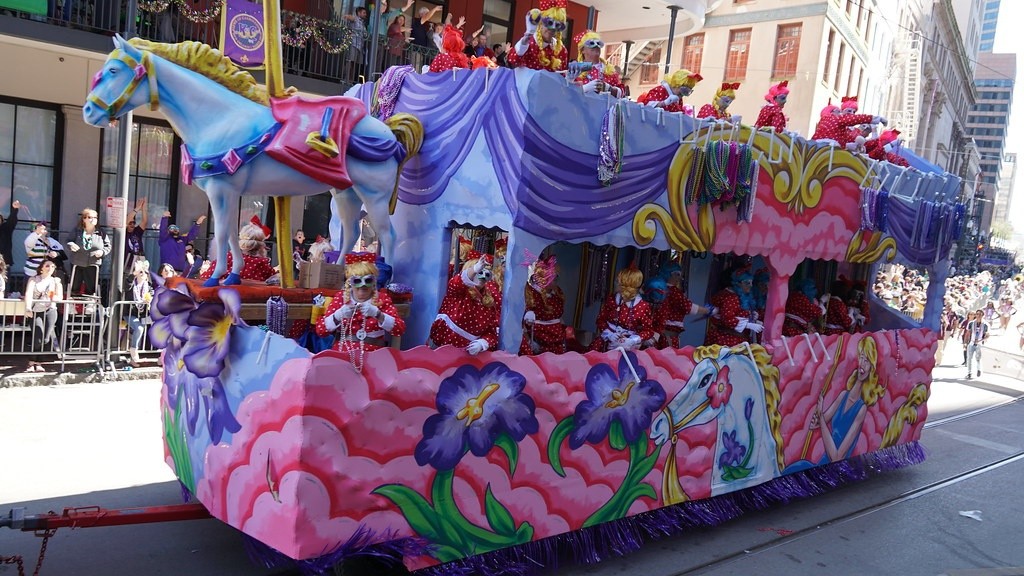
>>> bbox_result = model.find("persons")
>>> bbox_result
[518,248,572,356]
[703,266,771,347]
[335,0,442,85]
[0,200,112,348]
[430,0,790,132]
[316,251,405,352]
[199,217,275,281]
[425,232,509,356]
[782,280,828,337]
[585,262,654,351]
[643,264,719,349]
[872,232,1024,380]
[824,280,870,334]
[811,98,910,168]
[292,221,378,281]
[123,198,206,362]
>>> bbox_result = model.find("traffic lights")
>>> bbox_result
[978,244,983,250]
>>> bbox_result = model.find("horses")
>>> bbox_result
[83,31,423,284]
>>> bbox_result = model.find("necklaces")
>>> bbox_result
[858,188,967,262]
[616,299,633,329]
[818,293,831,333]
[684,140,758,226]
[340,305,365,373]
[598,83,624,186]
[369,65,415,121]
[266,297,287,336]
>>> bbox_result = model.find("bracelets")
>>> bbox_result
[133,210,138,214]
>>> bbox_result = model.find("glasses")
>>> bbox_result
[168,226,180,230]
[87,216,98,219]
[185,248,193,253]
[128,220,136,224]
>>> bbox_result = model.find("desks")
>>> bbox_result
[240,301,410,349]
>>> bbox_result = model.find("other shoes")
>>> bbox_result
[130,362,140,367]
[129,346,139,362]
[35,365,45,373]
[27,366,35,373]
[69,305,78,314]
[85,304,94,314]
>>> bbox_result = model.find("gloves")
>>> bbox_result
[665,95,678,106]
[880,118,888,125]
[732,114,743,121]
[621,338,636,347]
[821,295,828,302]
[712,307,719,316]
[607,331,621,342]
[845,142,858,150]
[745,319,765,333]
[849,308,854,313]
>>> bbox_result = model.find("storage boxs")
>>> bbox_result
[299,261,345,288]
